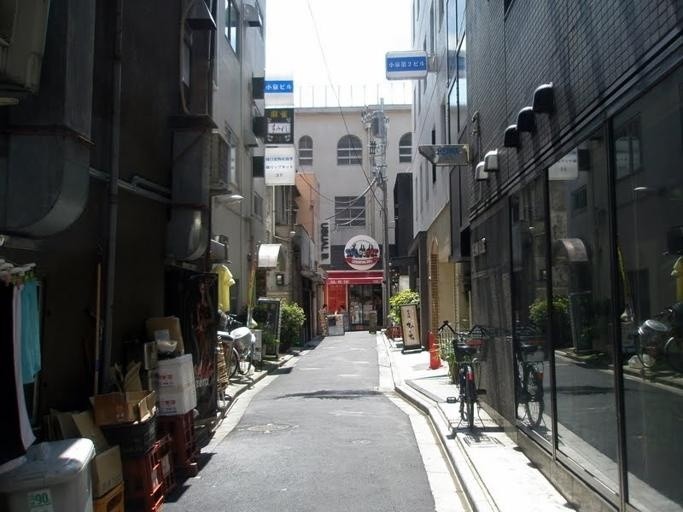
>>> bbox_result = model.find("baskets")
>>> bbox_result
[438,326,490,364]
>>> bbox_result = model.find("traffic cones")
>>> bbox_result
[426,331,446,371]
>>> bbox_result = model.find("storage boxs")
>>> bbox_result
[43,361,157,500]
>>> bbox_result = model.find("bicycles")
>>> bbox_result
[435,322,491,433]
[217,306,256,378]
[515,333,548,431]
[634,302,682,374]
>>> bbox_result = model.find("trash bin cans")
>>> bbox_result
[0,438,96,512]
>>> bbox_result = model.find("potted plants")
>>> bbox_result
[280,297,306,353]
